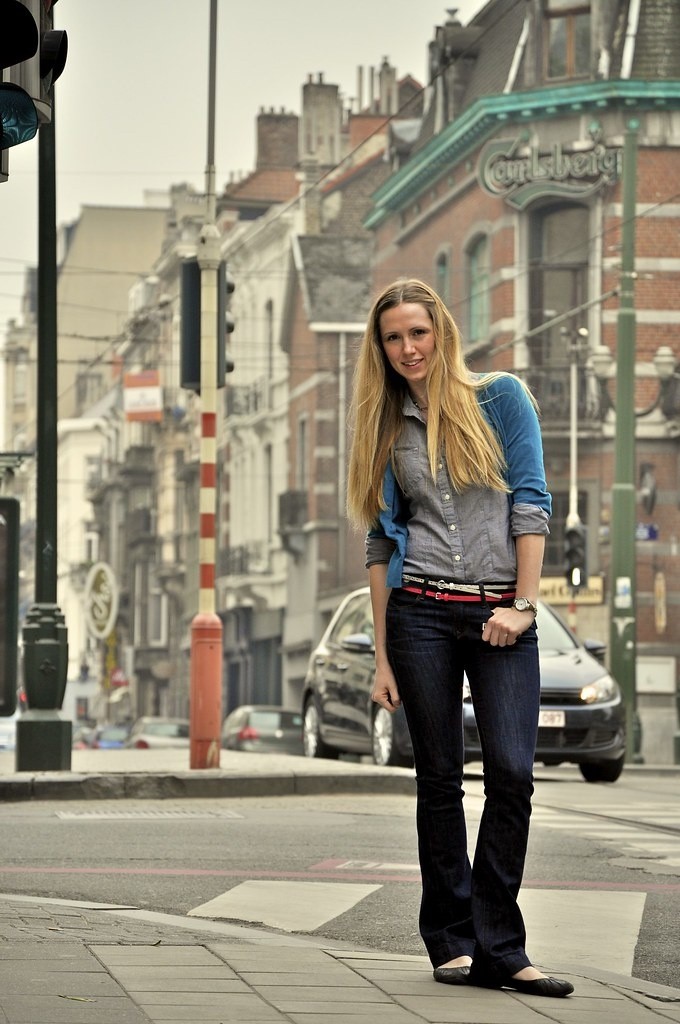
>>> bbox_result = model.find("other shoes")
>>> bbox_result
[505,973,575,997]
[432,965,470,985]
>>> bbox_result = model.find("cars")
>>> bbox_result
[127,715,197,749]
[218,703,314,755]
[305,580,630,785]
[94,730,128,748]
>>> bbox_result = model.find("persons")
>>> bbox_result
[347,280,573,996]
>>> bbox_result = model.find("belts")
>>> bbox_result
[402,573,517,600]
[402,586,515,603]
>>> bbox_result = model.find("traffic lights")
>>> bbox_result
[0,0,39,183]
[29,0,69,125]
[76,699,86,720]
[17,687,28,711]
[178,257,236,392]
[559,524,592,595]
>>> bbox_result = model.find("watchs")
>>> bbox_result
[514,598,537,614]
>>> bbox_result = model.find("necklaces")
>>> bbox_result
[412,399,428,411]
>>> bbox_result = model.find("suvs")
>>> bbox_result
[74,727,93,746]
